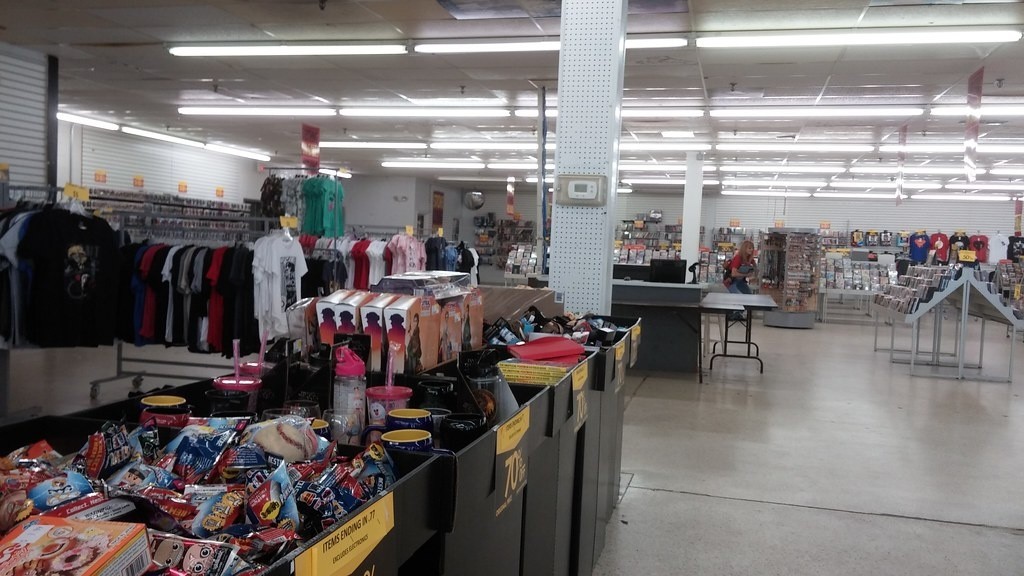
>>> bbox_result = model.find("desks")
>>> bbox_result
[701,292,779,374]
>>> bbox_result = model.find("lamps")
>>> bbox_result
[172,102,1024,118]
[164,24,1024,55]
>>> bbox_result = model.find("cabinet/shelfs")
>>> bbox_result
[818,261,1024,384]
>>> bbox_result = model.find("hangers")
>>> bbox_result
[12,183,92,218]
[265,215,294,242]
[133,225,257,253]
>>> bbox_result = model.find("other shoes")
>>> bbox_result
[727,315,746,321]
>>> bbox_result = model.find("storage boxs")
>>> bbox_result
[316,286,483,376]
[0,514,153,576]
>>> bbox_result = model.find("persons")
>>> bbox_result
[407,313,422,369]
[440,312,450,361]
[463,304,472,350]
[723,241,757,320]
